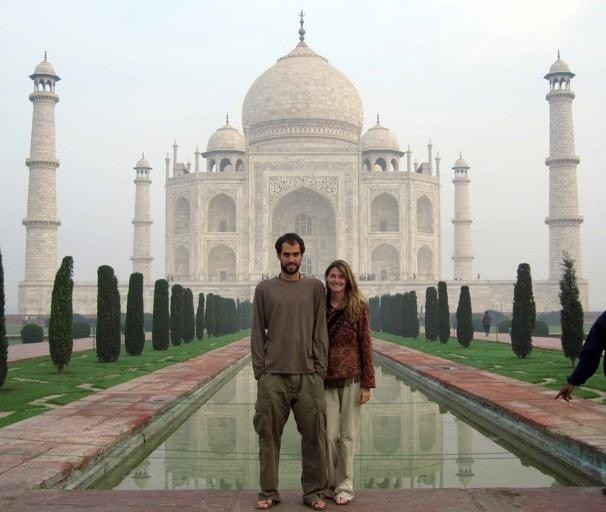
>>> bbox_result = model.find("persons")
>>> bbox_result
[484,310,491,335]
[320,259,375,504]
[250,231,334,511]
[553,309,606,403]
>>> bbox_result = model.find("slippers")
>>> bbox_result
[324,487,351,505]
[256,497,280,510]
[303,496,327,511]
